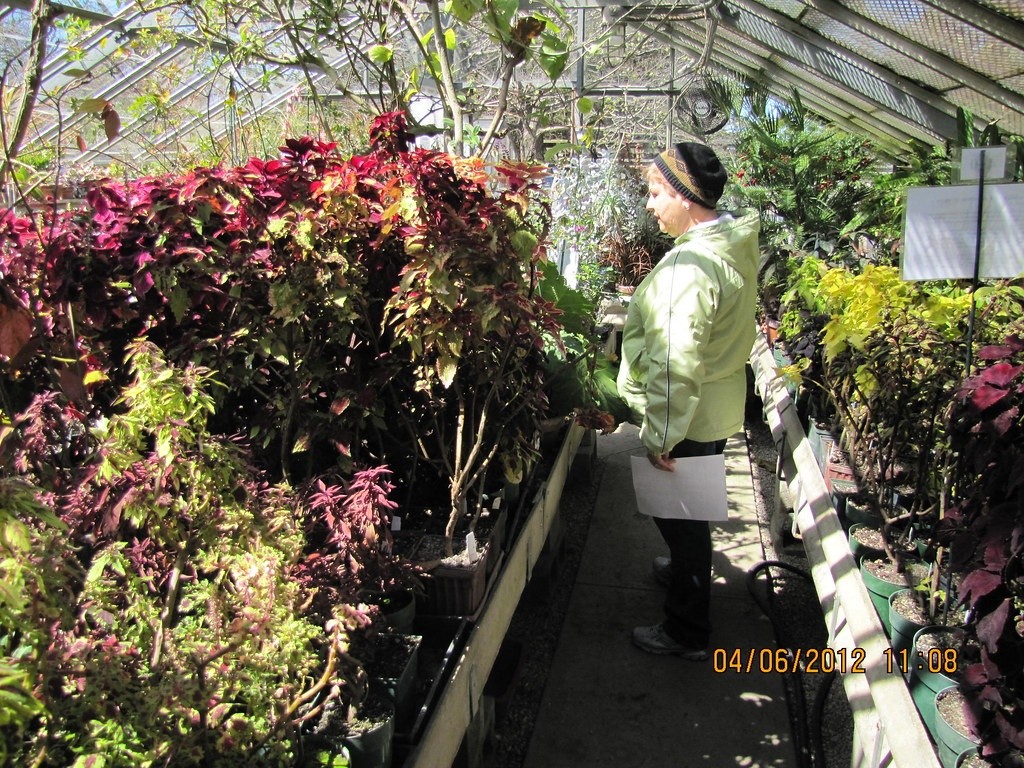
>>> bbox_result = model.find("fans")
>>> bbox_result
[676,78,731,135]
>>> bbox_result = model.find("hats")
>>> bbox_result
[654,142,727,208]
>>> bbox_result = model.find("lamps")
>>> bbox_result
[600,5,627,68]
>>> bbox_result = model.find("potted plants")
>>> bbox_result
[0,66,1024,768]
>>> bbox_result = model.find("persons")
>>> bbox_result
[616,143,762,659]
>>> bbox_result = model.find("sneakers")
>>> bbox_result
[653,556,676,586]
[631,621,708,660]
